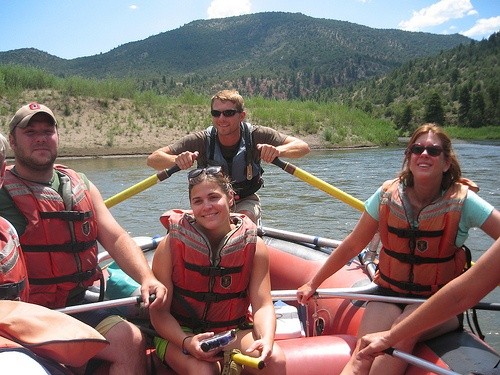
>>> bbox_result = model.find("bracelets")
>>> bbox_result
[182,336,196,356]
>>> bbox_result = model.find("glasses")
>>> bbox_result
[186,166,225,180]
[210,110,240,117]
[409,144,447,157]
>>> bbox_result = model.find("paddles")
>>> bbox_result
[312,291,500,311]
[55,294,156,315]
[104,163,180,209]
[384,346,462,375]
[258,148,475,266]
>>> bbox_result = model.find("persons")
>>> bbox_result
[147,89,310,240]
[0,130,31,303]
[150,167,286,375]
[356,234,500,360]
[296,122,500,375]
[1,102,168,375]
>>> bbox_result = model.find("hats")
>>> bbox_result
[8,102,59,133]
